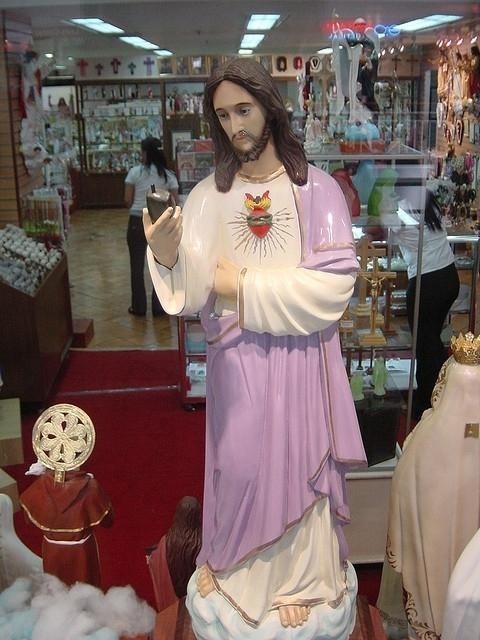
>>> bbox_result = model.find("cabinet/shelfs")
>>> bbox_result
[0,28,478,565]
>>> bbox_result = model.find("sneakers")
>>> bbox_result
[152,306,167,316]
[128,305,146,316]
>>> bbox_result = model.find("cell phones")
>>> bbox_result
[146,182,177,225]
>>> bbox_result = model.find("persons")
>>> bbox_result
[145,57,371,629]
[15,402,111,589]
[387,159,459,420]
[123,135,178,319]
[385,331,480,639]
[146,494,198,614]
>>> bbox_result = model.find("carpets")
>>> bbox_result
[2,349,413,614]
